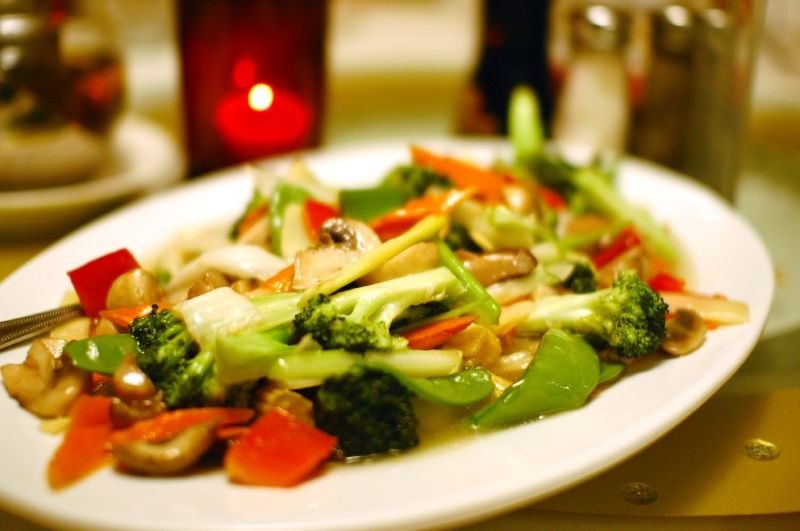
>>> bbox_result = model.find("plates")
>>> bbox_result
[1,108,189,243]
[0,134,777,531]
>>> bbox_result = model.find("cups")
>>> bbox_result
[174,0,328,174]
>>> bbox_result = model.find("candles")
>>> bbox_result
[215,83,314,163]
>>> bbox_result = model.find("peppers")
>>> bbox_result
[45,143,686,491]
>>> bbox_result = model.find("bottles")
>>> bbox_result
[452,0,699,172]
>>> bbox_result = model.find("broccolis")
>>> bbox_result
[130,87,669,461]
